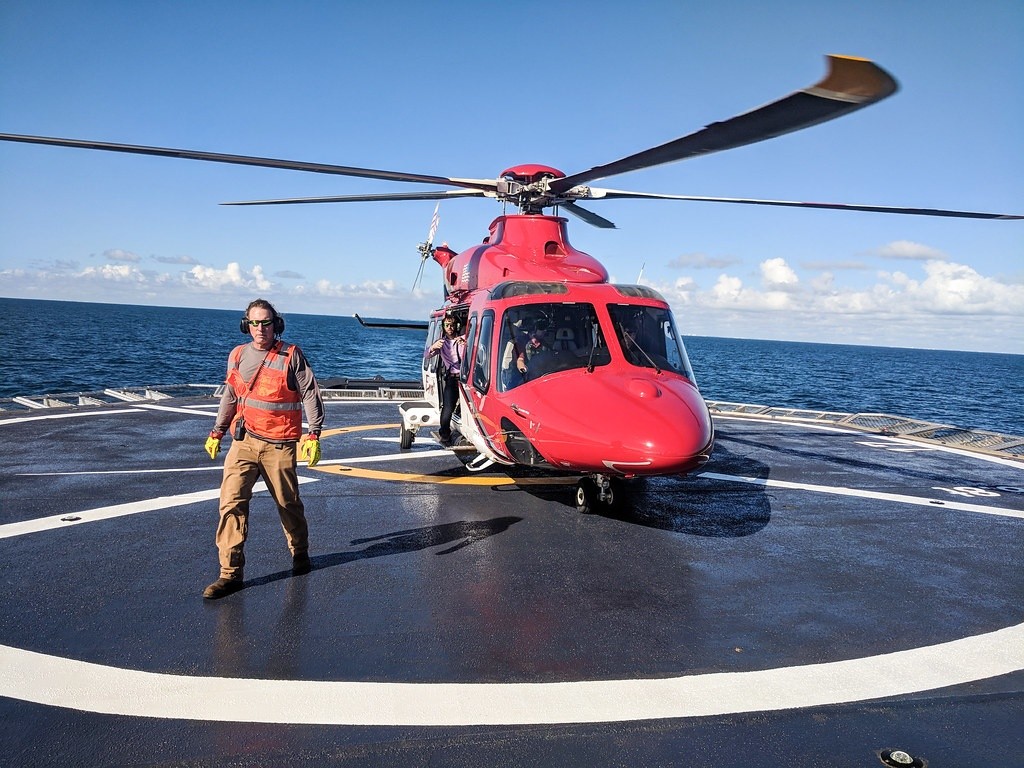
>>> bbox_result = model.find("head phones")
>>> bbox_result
[240,315,284,335]
[456,323,463,331]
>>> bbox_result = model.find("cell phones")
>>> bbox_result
[234,419,243,440]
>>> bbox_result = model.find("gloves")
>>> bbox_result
[301,430,321,466]
[205,431,223,459]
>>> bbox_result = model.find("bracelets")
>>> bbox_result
[309,430,321,435]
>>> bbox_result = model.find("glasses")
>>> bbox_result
[444,323,455,328]
[248,319,274,327]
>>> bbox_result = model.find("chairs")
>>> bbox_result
[552,327,578,351]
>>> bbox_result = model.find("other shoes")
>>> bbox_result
[203,578,243,598]
[442,435,450,444]
[292,550,310,576]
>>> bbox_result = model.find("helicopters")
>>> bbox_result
[1,51,1024,517]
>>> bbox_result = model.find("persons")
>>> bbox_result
[422,314,468,445]
[202,301,324,600]
[501,317,548,391]
[620,323,648,352]
